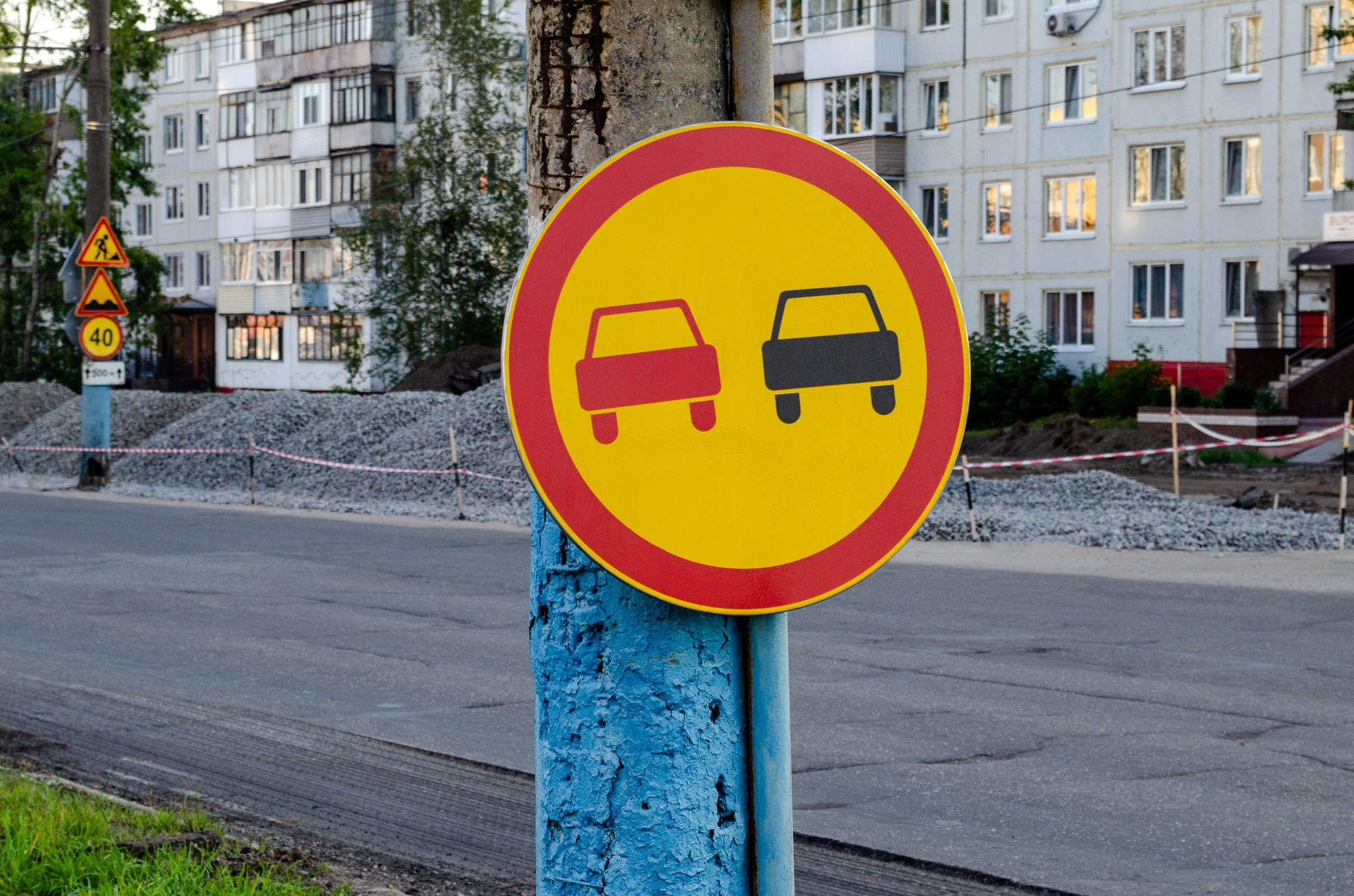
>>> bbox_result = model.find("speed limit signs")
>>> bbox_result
[78,314,123,361]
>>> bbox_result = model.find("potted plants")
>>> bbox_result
[1327,67,1354,110]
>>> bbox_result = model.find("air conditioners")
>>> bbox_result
[1045,13,1075,37]
